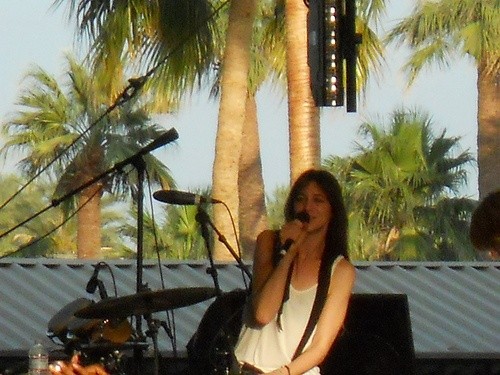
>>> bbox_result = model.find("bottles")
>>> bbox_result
[28,339,49,375]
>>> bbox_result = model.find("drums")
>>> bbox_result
[68,310,131,351]
[48,299,96,349]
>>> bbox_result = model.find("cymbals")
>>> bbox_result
[73,287,222,320]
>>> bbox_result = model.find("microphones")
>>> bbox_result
[153,190,221,205]
[86,263,101,294]
[280,211,310,255]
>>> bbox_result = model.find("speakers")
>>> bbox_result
[319,294,415,375]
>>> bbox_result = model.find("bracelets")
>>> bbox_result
[284,365,290,375]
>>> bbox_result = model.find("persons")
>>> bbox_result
[469,190,500,260]
[233,168,356,375]
[48,355,109,375]
